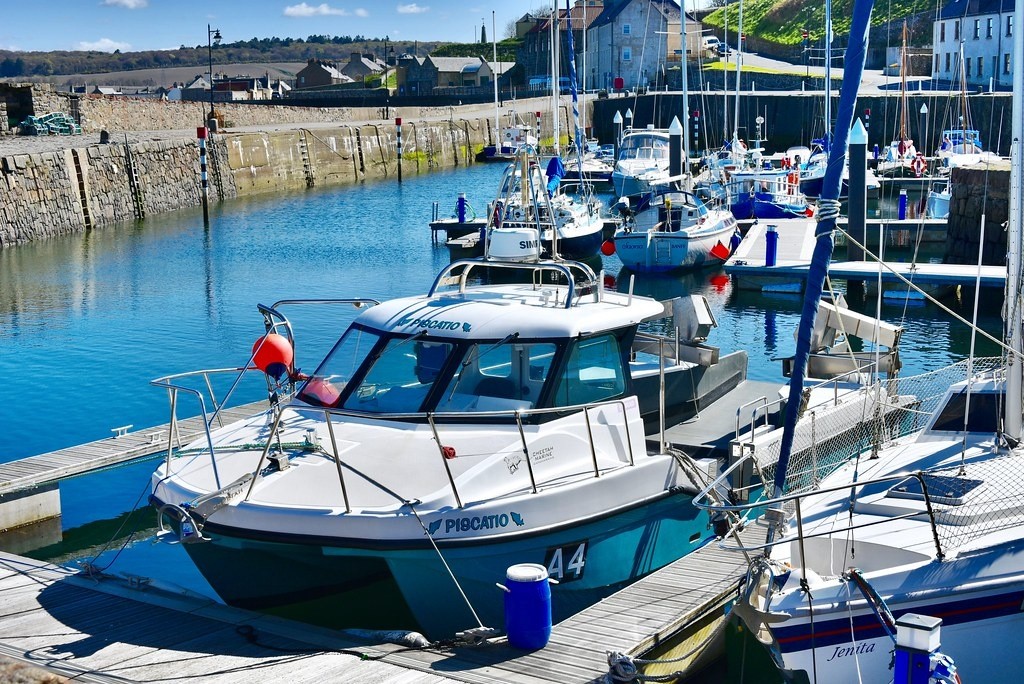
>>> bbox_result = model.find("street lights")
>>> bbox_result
[384,41,395,120]
[204,23,223,135]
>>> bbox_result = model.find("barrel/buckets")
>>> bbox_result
[496,563,559,650]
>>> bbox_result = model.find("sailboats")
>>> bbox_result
[483,11,537,162]
[147,0,1024,684]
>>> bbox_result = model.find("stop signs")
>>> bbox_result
[741,34,746,41]
[803,32,807,38]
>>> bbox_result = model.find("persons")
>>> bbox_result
[942,136,953,153]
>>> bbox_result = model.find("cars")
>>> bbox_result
[702,35,732,57]
